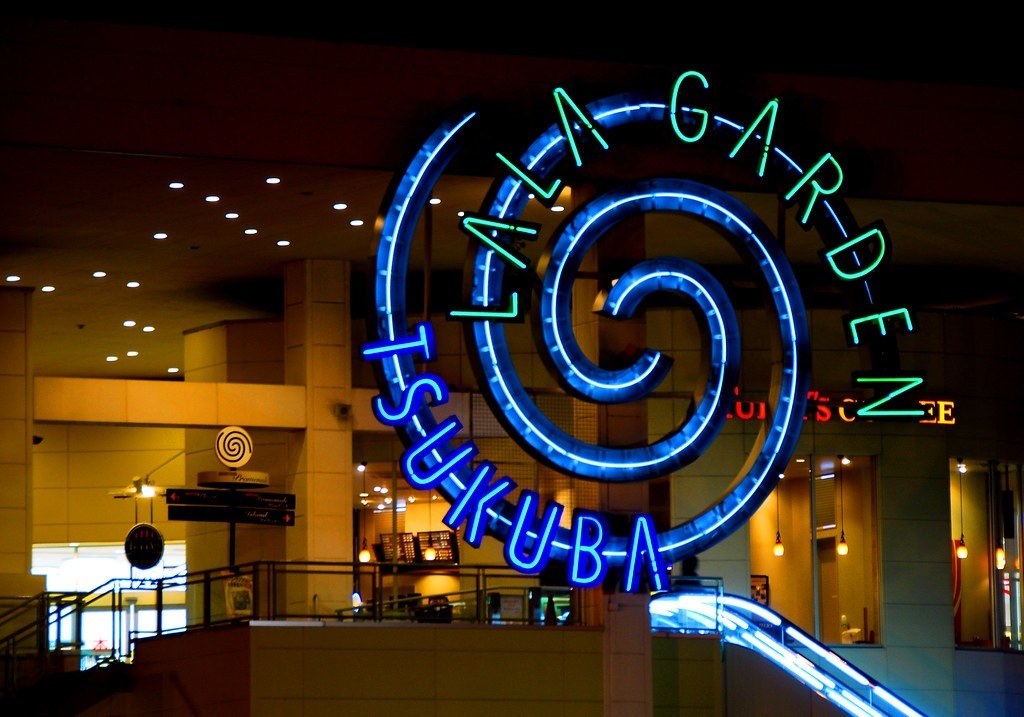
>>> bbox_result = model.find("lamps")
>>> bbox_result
[358,459,372,562]
[956,459,969,559]
[422,487,438,561]
[836,461,849,556]
[774,484,784,555]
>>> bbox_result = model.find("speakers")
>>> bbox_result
[335,403,352,416]
